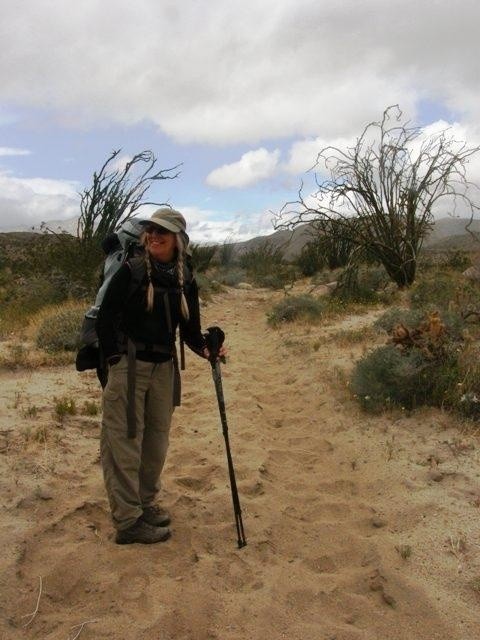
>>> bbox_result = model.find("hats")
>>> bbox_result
[117,218,144,248]
[137,208,187,236]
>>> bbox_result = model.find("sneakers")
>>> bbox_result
[143,506,171,527]
[114,519,172,545]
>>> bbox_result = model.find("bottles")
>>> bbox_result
[80,304,98,343]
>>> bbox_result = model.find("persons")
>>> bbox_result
[92,207,228,548]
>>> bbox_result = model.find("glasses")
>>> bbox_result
[142,225,173,238]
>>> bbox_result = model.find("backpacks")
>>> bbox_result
[75,253,192,439]
